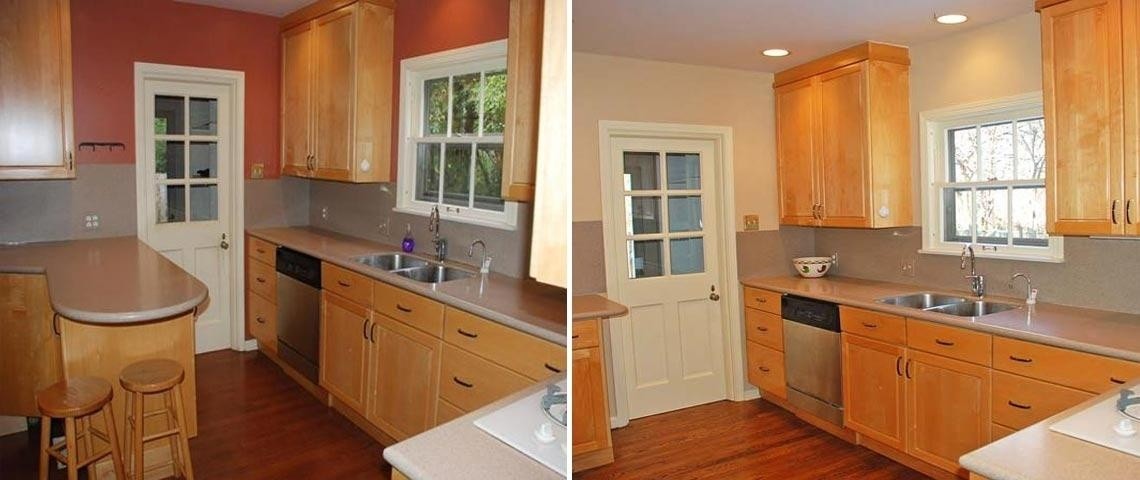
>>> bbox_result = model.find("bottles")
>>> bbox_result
[401,221,415,255]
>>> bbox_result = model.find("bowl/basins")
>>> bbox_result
[792,255,832,278]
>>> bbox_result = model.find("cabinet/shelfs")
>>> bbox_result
[320,262,444,452]
[244,233,276,352]
[437,306,566,427]
[771,40,916,230]
[835,305,993,480]
[1034,1,1138,236]
[0,0,75,181]
[275,2,395,186]
[742,282,785,399]
[574,296,631,473]
[989,334,1140,440]
[500,0,544,202]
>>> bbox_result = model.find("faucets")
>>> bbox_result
[429,202,448,261]
[1008,272,1039,305]
[467,239,491,274]
[465,274,485,302]
[959,243,985,295]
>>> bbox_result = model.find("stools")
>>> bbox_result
[118,358,196,479]
[34,374,124,480]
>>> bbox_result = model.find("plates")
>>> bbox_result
[541,391,567,429]
[1117,395,1139,423]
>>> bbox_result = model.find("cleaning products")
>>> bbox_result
[401,222,417,252]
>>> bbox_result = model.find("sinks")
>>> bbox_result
[873,289,972,309]
[922,298,1017,318]
[390,263,476,285]
[347,251,431,272]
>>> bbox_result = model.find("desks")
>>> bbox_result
[1,233,210,472]
